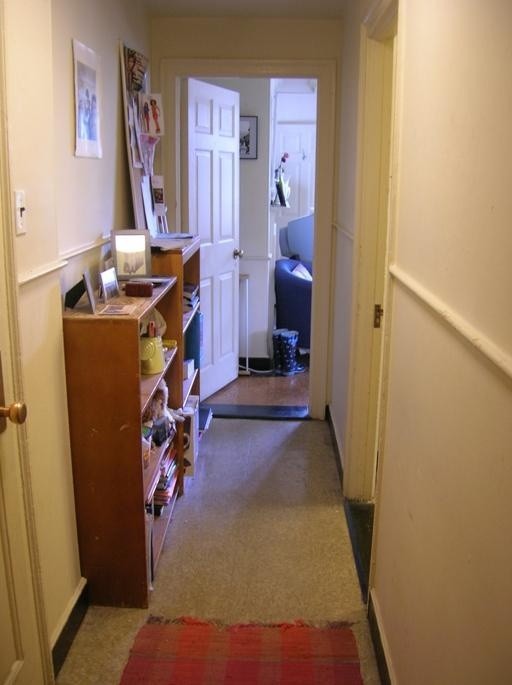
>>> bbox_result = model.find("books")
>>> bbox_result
[182,282,200,312]
[148,442,180,508]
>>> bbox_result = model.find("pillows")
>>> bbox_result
[291,263,312,282]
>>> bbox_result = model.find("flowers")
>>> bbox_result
[274,152,290,206]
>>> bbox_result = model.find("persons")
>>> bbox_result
[78,87,98,141]
[142,97,161,133]
[127,53,135,71]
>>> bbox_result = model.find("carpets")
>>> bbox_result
[120,614,365,684]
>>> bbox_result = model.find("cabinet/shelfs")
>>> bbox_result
[109,233,202,497]
[62,274,182,610]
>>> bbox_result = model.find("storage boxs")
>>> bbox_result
[179,393,201,478]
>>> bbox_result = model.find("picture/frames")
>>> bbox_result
[239,115,259,160]
[110,229,153,280]
[65,265,99,315]
[99,267,120,305]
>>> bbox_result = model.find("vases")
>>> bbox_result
[274,181,291,208]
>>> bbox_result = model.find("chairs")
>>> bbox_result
[275,259,312,347]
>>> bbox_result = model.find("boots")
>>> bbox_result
[273,329,287,374]
[281,331,305,375]
